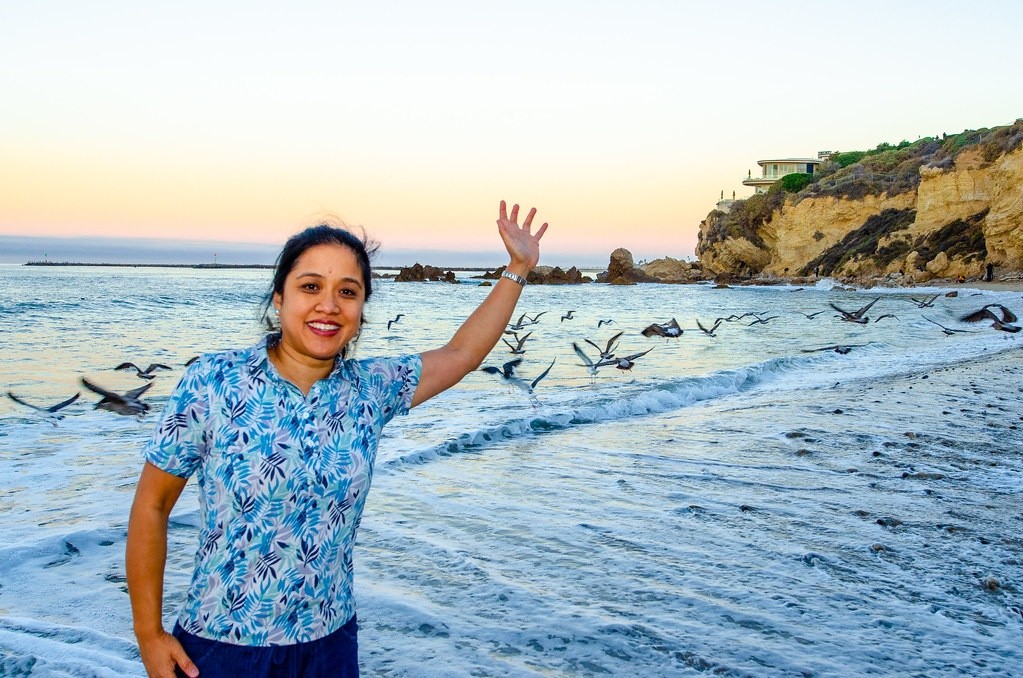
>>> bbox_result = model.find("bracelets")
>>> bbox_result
[501,271,527,286]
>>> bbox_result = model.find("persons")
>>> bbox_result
[125,199,548,678]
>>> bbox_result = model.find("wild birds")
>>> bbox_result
[7,356,200,421]
[388,314,405,330]
[476,293,1023,408]
